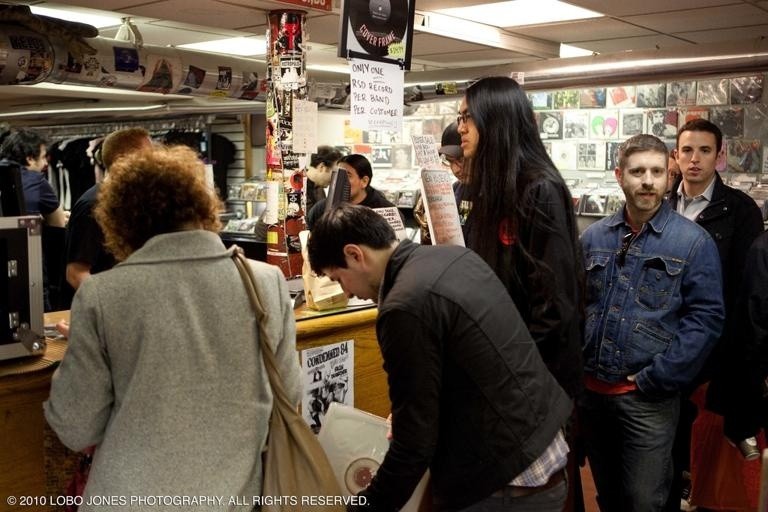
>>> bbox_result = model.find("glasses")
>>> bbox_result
[456,110,473,123]
[615,232,634,268]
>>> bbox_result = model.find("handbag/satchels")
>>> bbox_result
[261,404,346,512]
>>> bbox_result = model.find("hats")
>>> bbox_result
[439,122,463,158]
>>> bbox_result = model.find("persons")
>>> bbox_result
[573,134,725,511]
[67,126,154,307]
[308,154,406,232]
[304,146,342,208]
[665,119,764,510]
[726,227,765,447]
[438,123,475,232]
[0,129,65,312]
[456,76,588,512]
[41,147,304,512]
[306,202,576,512]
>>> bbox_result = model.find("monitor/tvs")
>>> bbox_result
[325,168,351,210]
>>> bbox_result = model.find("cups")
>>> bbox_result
[738,435,760,462]
